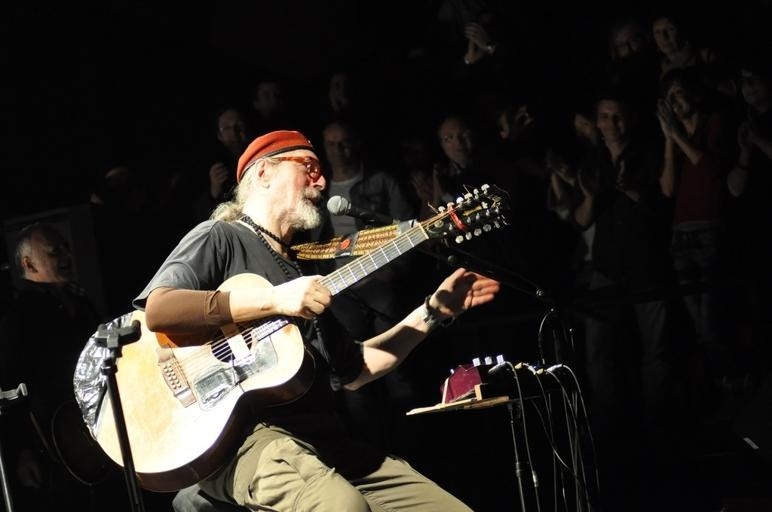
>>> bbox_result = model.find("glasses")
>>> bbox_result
[270,155,320,181]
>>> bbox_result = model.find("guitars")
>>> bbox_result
[73,183,510,492]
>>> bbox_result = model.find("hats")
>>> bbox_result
[235,128,316,184]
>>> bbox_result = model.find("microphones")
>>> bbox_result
[327,193,397,227]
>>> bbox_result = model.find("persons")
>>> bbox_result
[1,0,770,512]
